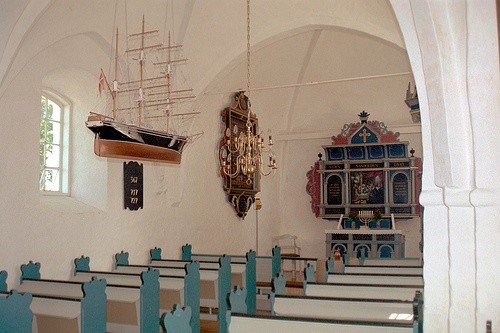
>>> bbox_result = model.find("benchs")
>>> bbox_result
[0,242,424,333]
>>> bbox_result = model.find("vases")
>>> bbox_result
[352,221,355,230]
[376,221,380,230]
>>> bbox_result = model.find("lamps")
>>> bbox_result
[219,0,277,184]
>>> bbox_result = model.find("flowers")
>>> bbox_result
[370,209,383,223]
[348,209,359,222]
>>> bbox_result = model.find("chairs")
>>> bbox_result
[272,234,301,280]
